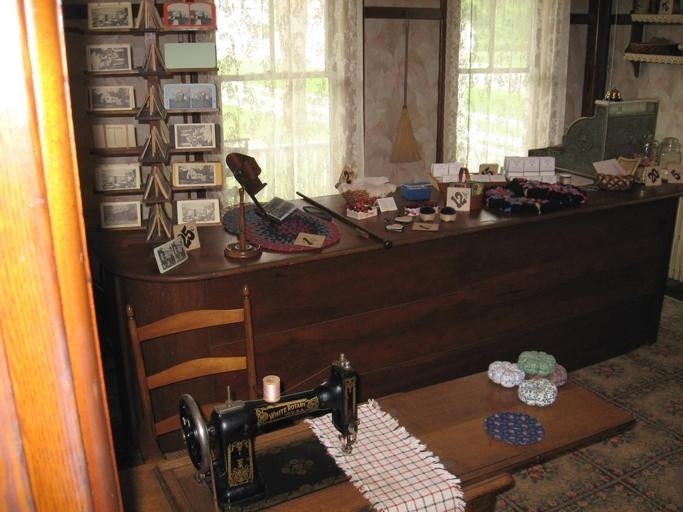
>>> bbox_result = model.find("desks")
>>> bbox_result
[117,371,637,511]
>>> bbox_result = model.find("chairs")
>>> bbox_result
[126,284,264,464]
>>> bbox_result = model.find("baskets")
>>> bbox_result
[596,173,634,192]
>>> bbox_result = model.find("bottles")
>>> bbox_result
[634,134,659,184]
[659,137,681,184]
